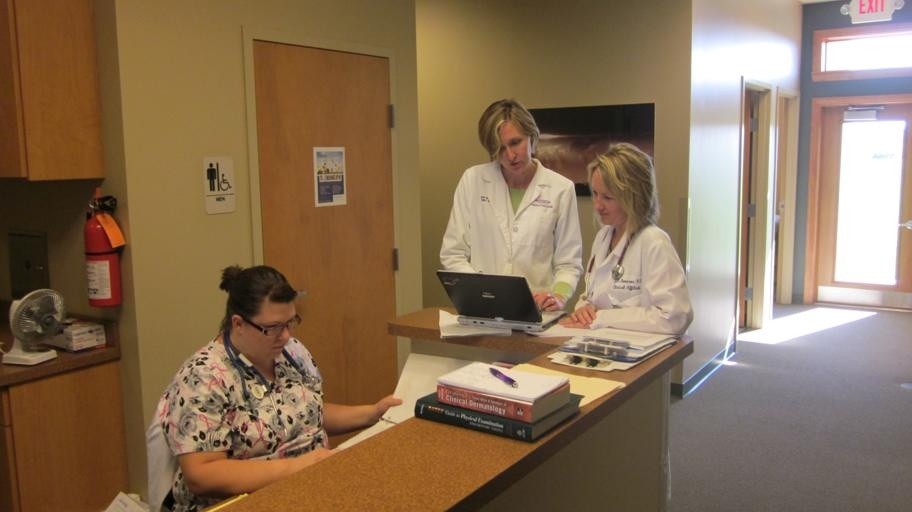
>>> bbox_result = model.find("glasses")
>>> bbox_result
[241,313,302,337]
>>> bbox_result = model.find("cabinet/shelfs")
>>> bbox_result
[1,0,106,182]
[1,312,128,512]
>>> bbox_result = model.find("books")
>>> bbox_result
[415,360,585,441]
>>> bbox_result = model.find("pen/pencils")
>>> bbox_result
[490,368,518,388]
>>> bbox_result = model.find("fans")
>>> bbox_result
[2,288,64,365]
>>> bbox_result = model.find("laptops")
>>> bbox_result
[436,268,568,332]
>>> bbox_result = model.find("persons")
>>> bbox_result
[144,266,402,511]
[440,97,585,312]
[557,143,694,335]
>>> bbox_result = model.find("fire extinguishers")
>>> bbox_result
[83,195,123,308]
